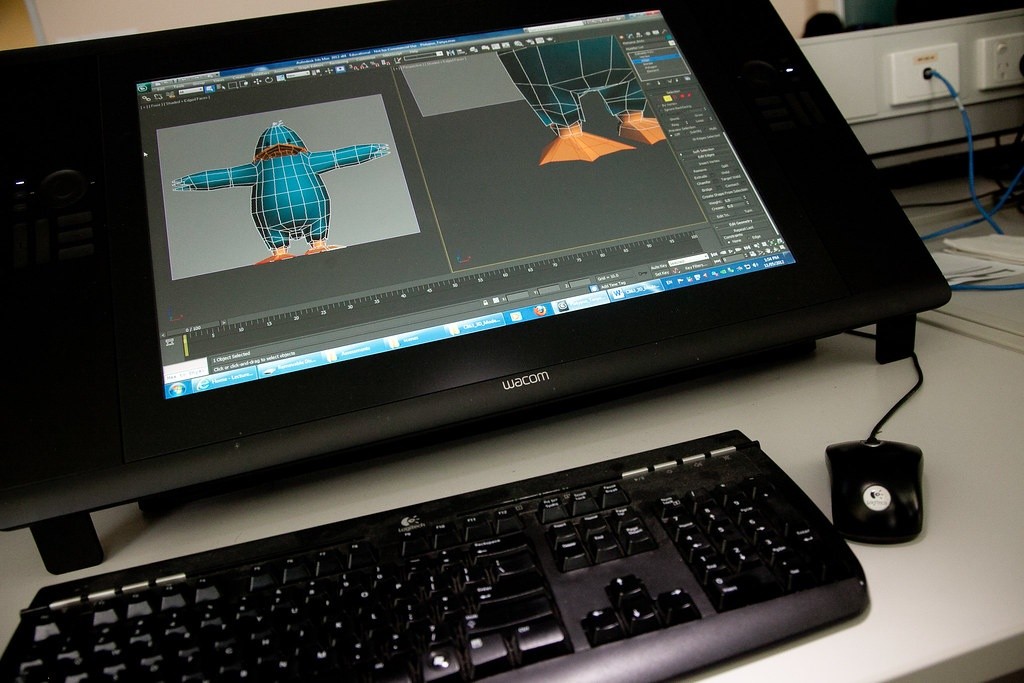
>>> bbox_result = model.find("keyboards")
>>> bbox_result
[0,430,869,683]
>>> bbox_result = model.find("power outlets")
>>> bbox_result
[889,43,960,106]
[976,33,1024,90]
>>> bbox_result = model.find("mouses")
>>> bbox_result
[824,434,924,547]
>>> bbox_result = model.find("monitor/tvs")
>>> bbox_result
[0,0,953,577]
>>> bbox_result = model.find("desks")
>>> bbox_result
[0,177,1024,683]
